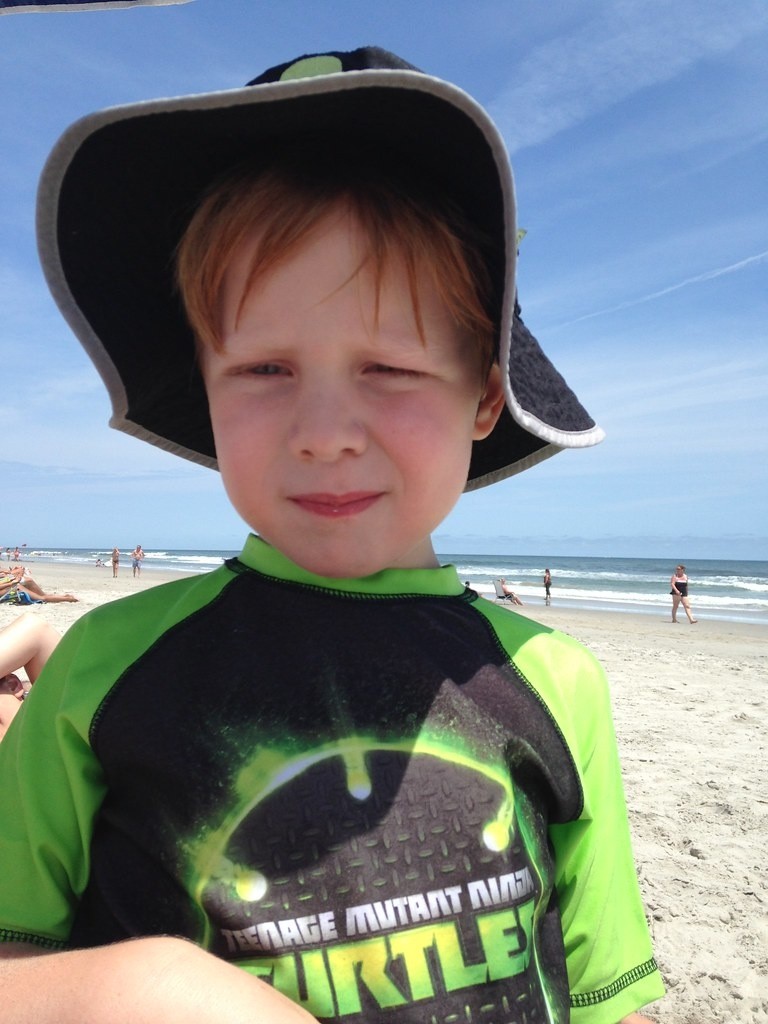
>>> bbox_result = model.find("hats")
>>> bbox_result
[36,46,606,493]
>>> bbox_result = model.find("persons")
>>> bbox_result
[670,565,697,625]
[543,569,551,600]
[131,545,144,578]
[0,547,79,744]
[501,578,523,606]
[95,559,101,567]
[0,48,667,1024]
[112,547,120,578]
